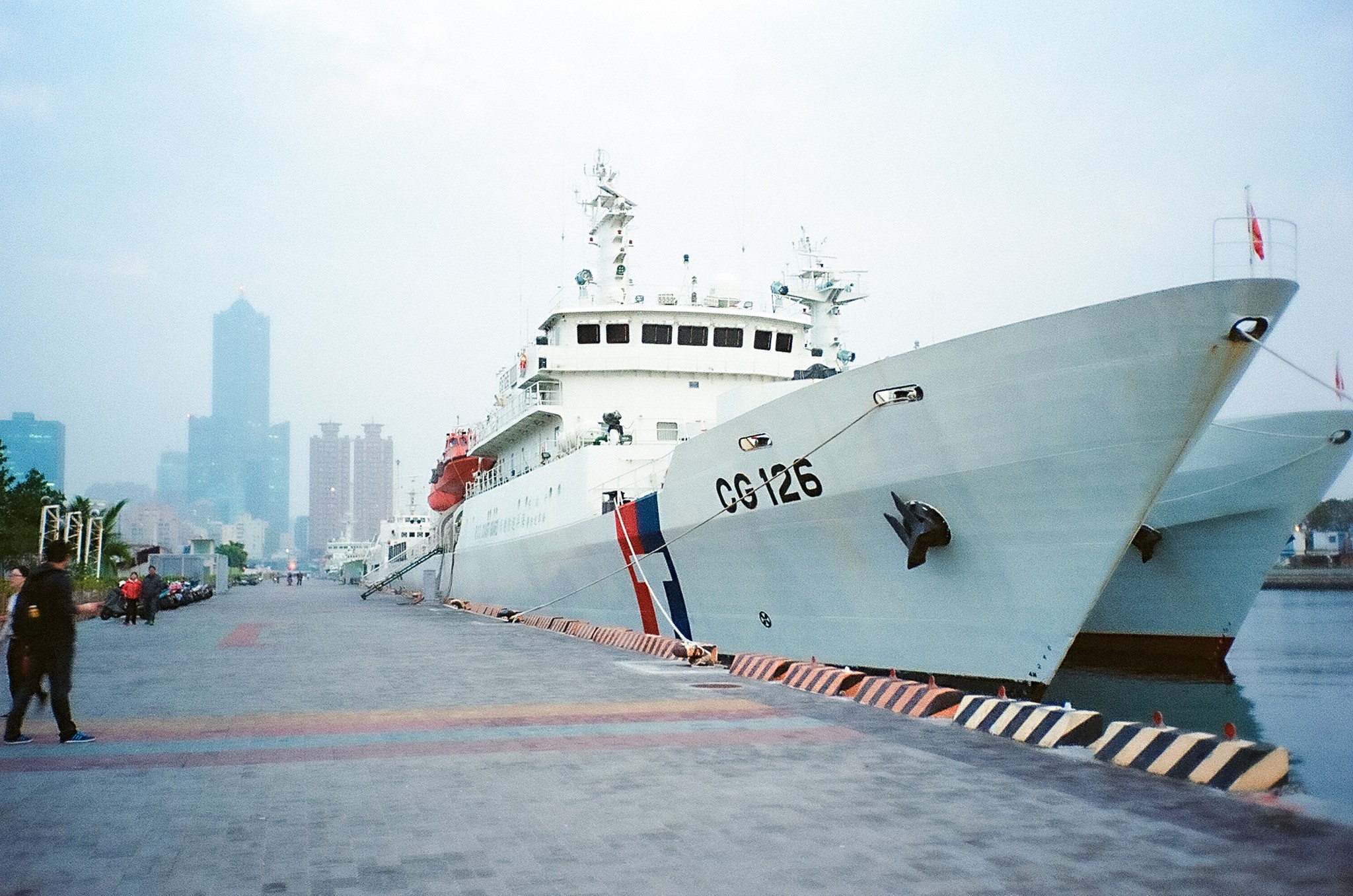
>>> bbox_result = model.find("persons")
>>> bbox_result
[277,573,279,583]
[0,566,48,720]
[287,573,292,586]
[3,538,105,746]
[122,572,143,625]
[272,574,277,580]
[142,565,162,626]
[296,571,303,586]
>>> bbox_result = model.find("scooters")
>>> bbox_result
[287,577,292,586]
[101,574,215,621]
[273,576,277,583]
[227,574,264,587]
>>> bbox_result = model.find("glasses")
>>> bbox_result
[9,574,25,578]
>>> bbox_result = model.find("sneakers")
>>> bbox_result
[61,732,96,743]
[4,734,33,744]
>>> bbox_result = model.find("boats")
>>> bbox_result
[359,160,1305,705]
[426,481,460,514]
[430,455,495,498]
[1077,406,1353,679]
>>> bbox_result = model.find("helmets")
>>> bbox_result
[118,580,125,588]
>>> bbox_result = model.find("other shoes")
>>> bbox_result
[144,622,150,624]
[0,712,11,719]
[124,621,129,625]
[38,691,48,706]
[149,621,153,625]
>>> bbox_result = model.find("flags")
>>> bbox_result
[1336,366,1346,399]
[1247,204,1264,260]
